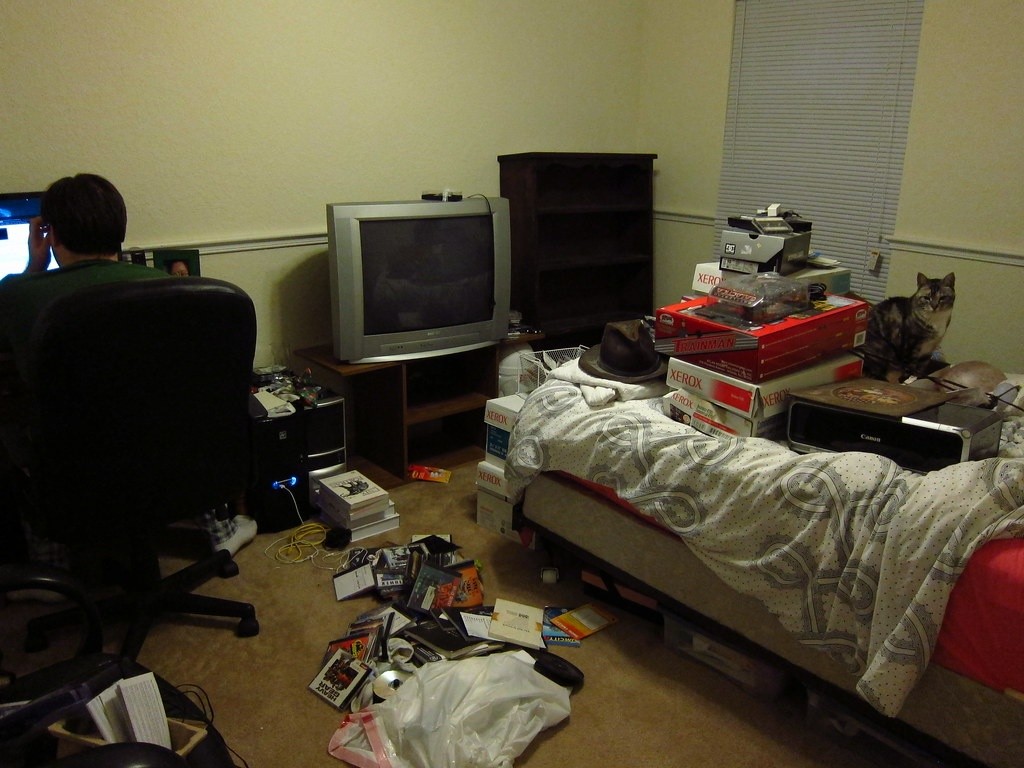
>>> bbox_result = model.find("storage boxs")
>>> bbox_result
[484,394,527,467]
[690,261,851,297]
[46,716,209,761]
[476,462,546,551]
[654,295,870,380]
[664,356,864,440]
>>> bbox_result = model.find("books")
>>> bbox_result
[307,532,618,711]
[314,469,399,542]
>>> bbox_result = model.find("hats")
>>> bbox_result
[579,319,668,383]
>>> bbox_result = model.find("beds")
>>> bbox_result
[502,357,1024,768]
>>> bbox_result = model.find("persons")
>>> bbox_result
[0,174,128,293]
[169,260,190,278]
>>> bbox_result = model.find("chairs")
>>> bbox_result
[0,277,260,768]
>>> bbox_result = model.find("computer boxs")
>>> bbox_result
[249,405,309,534]
[306,392,349,514]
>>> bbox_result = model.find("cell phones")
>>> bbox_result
[448,195,462,201]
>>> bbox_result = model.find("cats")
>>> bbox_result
[863,272,957,383]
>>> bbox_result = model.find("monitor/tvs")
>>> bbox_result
[0,191,60,285]
[326,197,511,363]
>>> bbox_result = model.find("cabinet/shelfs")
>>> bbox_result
[496,152,658,350]
[295,331,547,479]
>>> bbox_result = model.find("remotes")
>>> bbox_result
[421,194,443,200]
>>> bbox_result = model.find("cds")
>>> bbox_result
[372,668,413,703]
